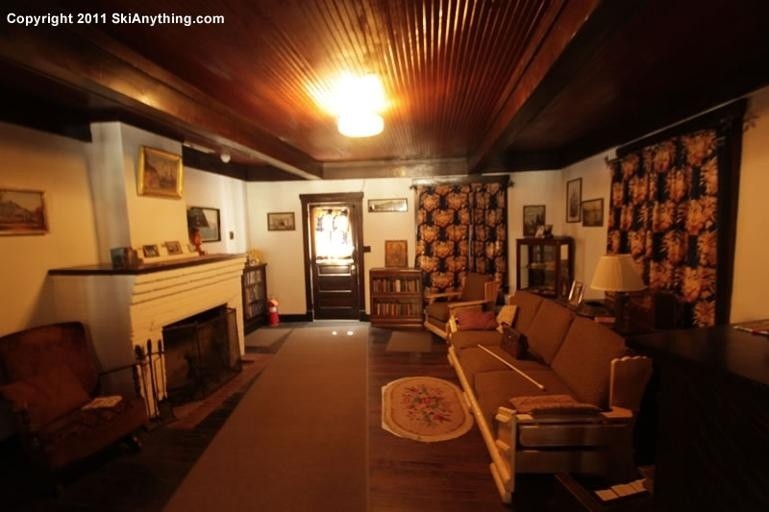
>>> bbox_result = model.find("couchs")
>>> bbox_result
[448,289,653,504]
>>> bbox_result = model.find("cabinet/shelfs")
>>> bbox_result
[241,260,271,336]
[369,269,424,330]
[516,236,576,301]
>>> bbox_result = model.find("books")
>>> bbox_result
[244,270,269,319]
[372,277,423,318]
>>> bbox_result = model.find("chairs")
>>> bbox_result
[0,321,155,504]
[425,272,498,344]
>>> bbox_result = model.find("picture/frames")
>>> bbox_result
[188,207,221,245]
[368,199,406,212]
[568,279,586,305]
[582,197,604,226]
[385,240,408,269]
[0,188,51,238]
[133,146,185,199]
[268,211,297,233]
[566,177,583,225]
[523,204,547,238]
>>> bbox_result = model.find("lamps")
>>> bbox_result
[588,253,647,334]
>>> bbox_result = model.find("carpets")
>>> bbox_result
[380,375,473,443]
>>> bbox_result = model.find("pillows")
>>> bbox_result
[509,393,602,415]
[457,308,498,331]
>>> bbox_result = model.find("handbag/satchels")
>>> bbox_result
[500,321,530,360]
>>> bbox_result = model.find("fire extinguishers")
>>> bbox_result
[266,299,279,327]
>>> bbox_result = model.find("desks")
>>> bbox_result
[626,316,769,512]
